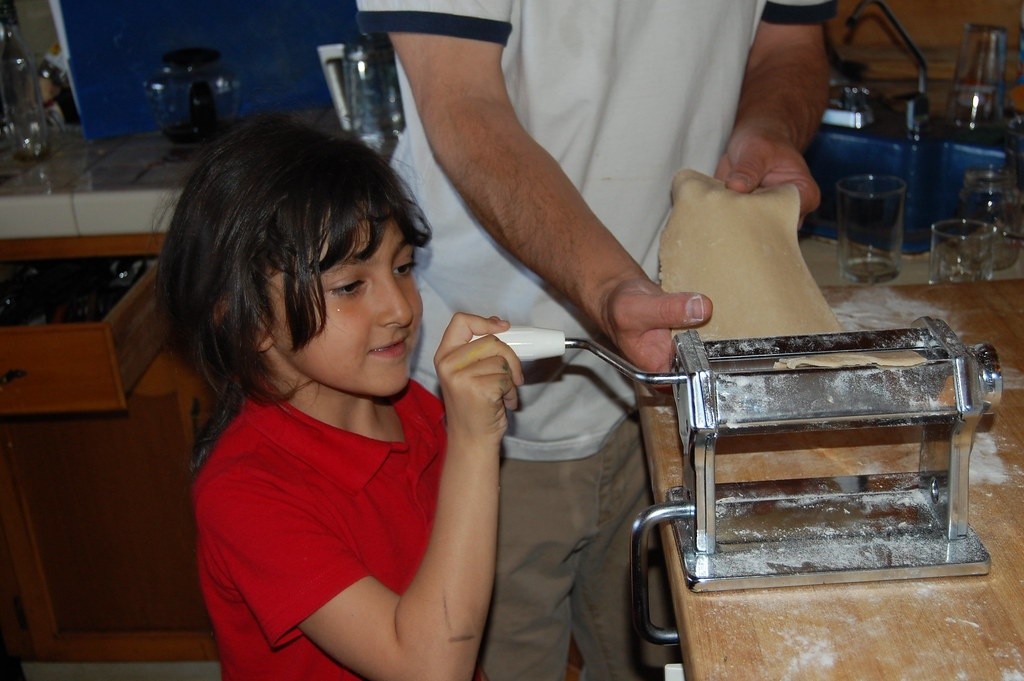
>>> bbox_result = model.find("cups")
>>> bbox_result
[928,220,996,285]
[833,173,907,283]
[951,22,1009,135]
[341,31,404,146]
[961,169,1019,270]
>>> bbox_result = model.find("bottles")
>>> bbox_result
[0,0,50,160]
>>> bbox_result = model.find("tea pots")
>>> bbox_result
[146,47,241,141]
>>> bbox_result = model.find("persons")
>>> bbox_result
[358,0,840,681]
[147,111,525,681]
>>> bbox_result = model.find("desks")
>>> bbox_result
[0,104,398,666]
[629,226,1024,681]
